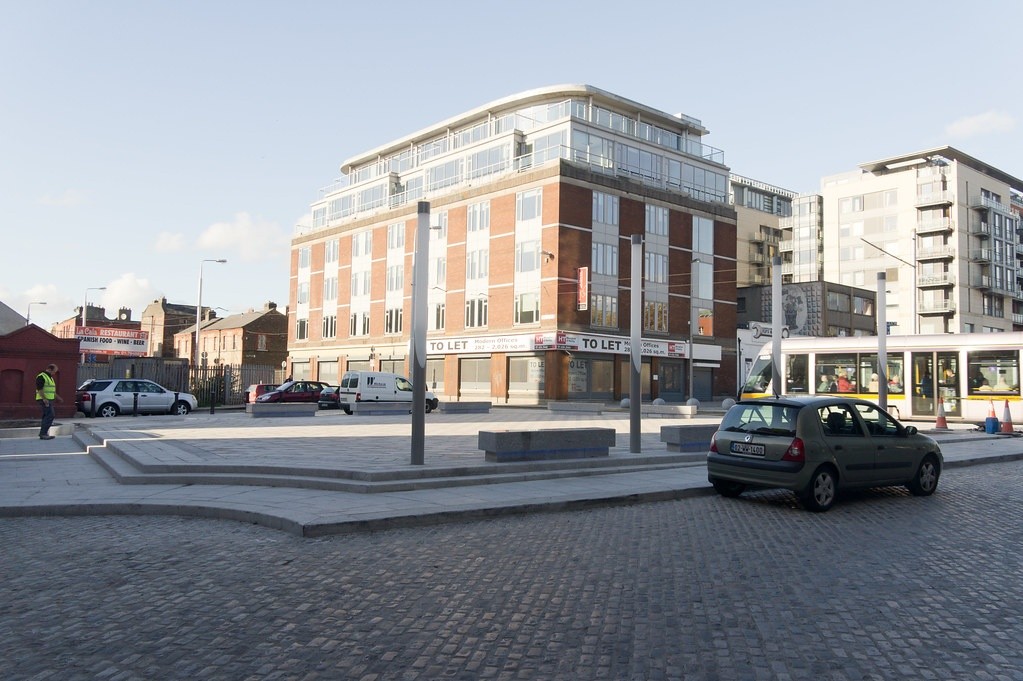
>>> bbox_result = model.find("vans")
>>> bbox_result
[339,370,438,415]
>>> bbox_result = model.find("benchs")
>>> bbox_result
[252,403,318,418]
[438,402,493,414]
[642,405,698,419]
[548,402,605,416]
[350,402,412,416]
[661,424,720,452]
[478,428,617,464]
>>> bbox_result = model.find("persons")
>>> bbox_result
[946,369,956,384]
[284,375,293,390]
[817,372,899,392]
[969,372,1009,390]
[920,371,934,411]
[35,364,64,439]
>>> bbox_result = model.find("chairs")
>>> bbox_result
[825,412,852,434]
[740,408,800,429]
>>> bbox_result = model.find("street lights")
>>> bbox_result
[82,286,106,363]
[194,258,226,390]
[26,302,47,326]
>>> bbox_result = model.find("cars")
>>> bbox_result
[318,385,341,409]
[707,395,945,511]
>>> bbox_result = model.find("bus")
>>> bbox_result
[740,330,1023,425]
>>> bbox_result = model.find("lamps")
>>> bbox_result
[690,258,701,264]
[540,250,555,264]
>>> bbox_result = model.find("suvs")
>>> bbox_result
[74,377,198,418]
[255,381,331,403]
[245,384,282,402]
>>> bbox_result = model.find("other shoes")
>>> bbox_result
[40,434,55,440]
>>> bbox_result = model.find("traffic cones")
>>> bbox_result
[986,398,995,417]
[930,396,952,430]
[994,398,1019,435]
